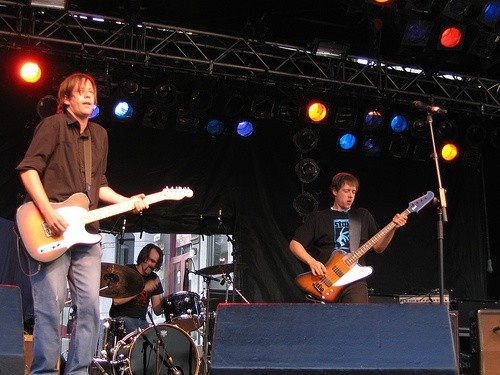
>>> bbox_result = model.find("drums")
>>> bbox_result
[161,290,205,334]
[112,324,200,375]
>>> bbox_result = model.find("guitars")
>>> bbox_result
[13,185,194,264]
[295,191,434,303]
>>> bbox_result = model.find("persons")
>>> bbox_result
[15,73,149,375]
[289,172,408,303]
[109,243,164,343]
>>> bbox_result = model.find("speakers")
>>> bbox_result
[0,285,25,375]
[210,302,458,375]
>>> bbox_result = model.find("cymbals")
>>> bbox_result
[196,261,254,276]
[98,263,145,299]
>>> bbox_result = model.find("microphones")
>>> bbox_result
[217,209,222,230]
[119,216,127,244]
[184,260,189,291]
[412,101,447,114]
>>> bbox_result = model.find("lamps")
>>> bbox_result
[293,129,320,216]
